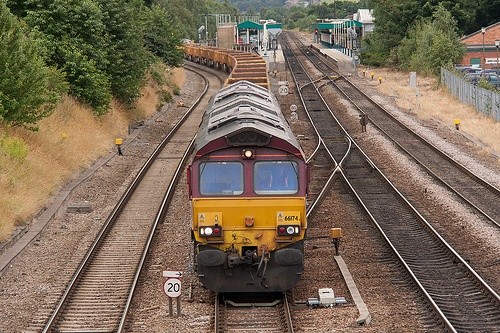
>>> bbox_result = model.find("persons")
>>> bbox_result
[267,163,289,188]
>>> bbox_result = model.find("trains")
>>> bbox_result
[178,39,310,308]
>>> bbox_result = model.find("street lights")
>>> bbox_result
[481,26,485,75]
[495,39,500,70]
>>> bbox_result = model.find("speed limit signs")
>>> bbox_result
[278,86,288,96]
[163,278,181,298]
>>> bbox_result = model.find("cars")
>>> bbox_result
[456,67,500,86]
